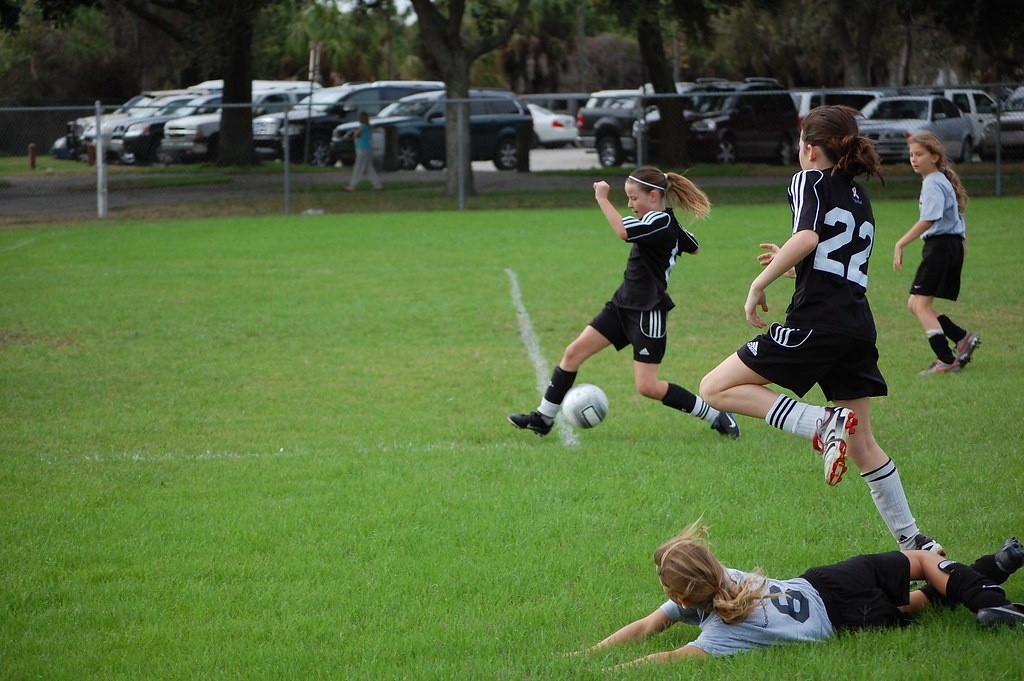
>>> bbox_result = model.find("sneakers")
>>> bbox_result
[711,411,741,442]
[906,533,946,586]
[812,407,858,486]
[953,331,982,368]
[918,358,961,377]
[508,411,554,437]
[977,603,1024,628]
[993,537,1024,573]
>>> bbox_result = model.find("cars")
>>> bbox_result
[576,78,1024,168]
[855,96,977,165]
[527,102,578,150]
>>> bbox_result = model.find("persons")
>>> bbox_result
[892,130,982,378]
[550,509,1024,675]
[340,111,386,192]
[506,165,742,443]
[699,104,947,586]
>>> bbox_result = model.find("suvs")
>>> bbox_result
[519,91,591,119]
[50,77,326,164]
[328,86,536,172]
[633,76,801,165]
[252,81,446,173]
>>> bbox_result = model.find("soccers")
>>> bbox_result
[561,384,609,430]
